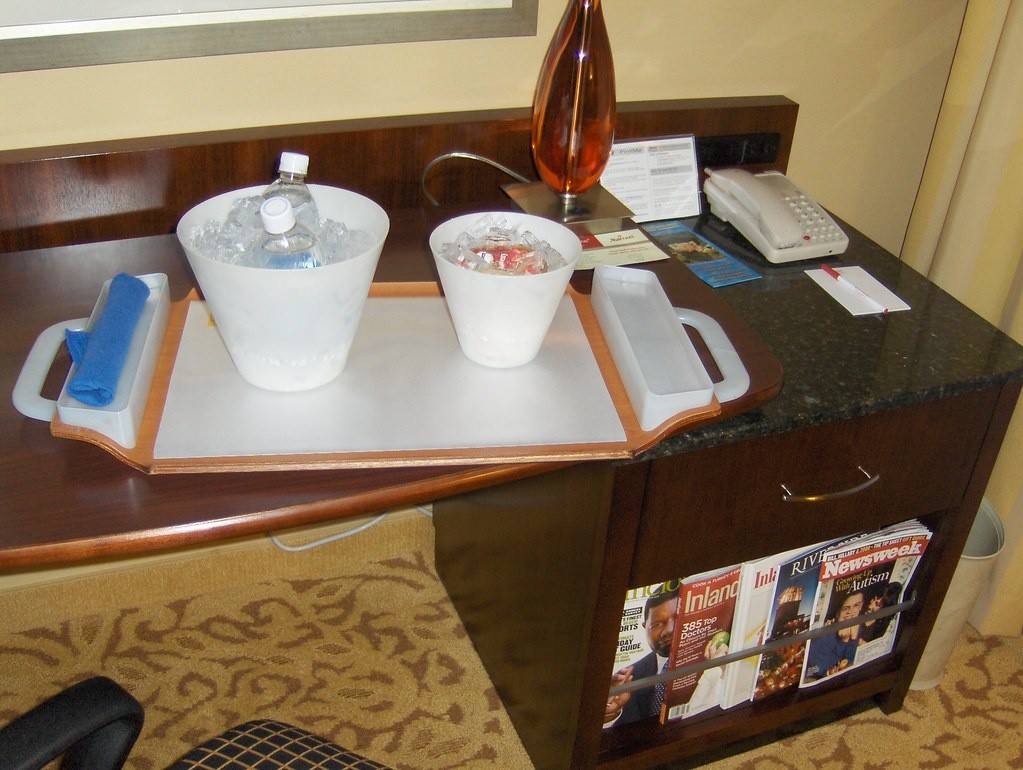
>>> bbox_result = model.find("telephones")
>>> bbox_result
[704,164,850,265]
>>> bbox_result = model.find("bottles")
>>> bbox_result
[256,197,326,267]
[262,152,321,231]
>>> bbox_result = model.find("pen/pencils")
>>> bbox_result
[820,259,887,315]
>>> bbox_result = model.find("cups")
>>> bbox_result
[427,210,583,368]
[177,182,391,393]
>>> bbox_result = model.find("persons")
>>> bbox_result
[603,580,680,728]
[688,639,728,712]
[859,583,902,644]
[803,593,863,683]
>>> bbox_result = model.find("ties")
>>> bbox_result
[651,659,670,717]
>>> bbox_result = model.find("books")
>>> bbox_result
[604,519,932,730]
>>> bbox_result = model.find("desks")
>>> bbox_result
[0,92,1023,770]
